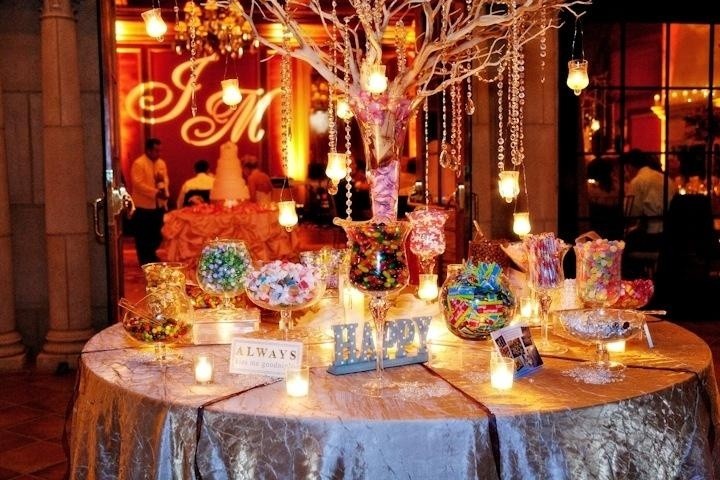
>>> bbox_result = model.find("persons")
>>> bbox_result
[334,159,371,221]
[661,150,687,190]
[241,154,274,200]
[584,161,622,206]
[132,137,169,266]
[175,159,216,209]
[624,148,676,231]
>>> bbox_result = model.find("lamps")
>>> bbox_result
[363,65,387,97]
[221,79,242,109]
[568,59,589,95]
[491,357,514,390]
[514,213,531,238]
[326,152,347,183]
[279,201,297,231]
[418,274,438,303]
[498,170,520,203]
[142,6,168,42]
[193,355,213,382]
[286,368,308,395]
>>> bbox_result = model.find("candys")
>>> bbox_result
[442,263,515,338]
[569,313,633,338]
[199,239,250,298]
[528,233,568,297]
[124,312,191,342]
[407,208,446,258]
[620,279,654,307]
[511,235,568,262]
[144,262,186,316]
[303,246,342,288]
[246,260,323,307]
[576,238,623,304]
[346,216,409,290]
[469,236,507,268]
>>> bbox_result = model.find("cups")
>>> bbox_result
[117,294,195,371]
[403,212,450,275]
[559,309,645,384]
[418,274,438,299]
[143,263,194,327]
[299,248,348,297]
[573,242,624,309]
[286,365,309,397]
[195,239,255,320]
[244,275,328,341]
[193,353,214,384]
[490,358,513,391]
[520,296,535,318]
[333,217,413,400]
[524,236,571,355]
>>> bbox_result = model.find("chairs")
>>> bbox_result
[184,189,209,205]
[600,195,635,226]
[661,195,715,288]
